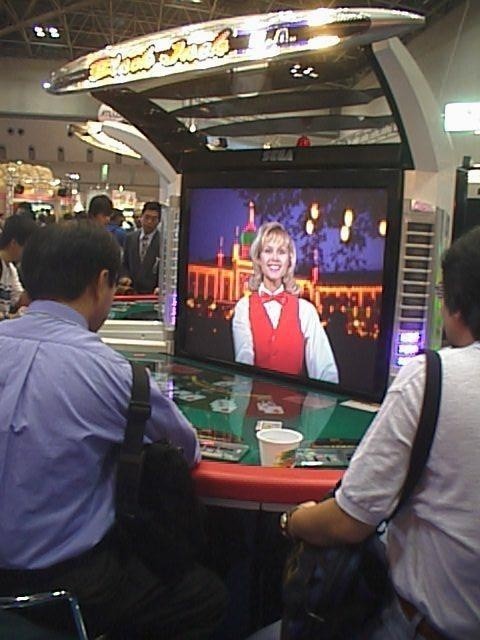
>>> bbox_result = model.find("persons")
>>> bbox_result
[110,209,125,227]
[86,194,124,247]
[241,223,480,639]
[13,201,86,227]
[0,214,37,319]
[0,220,227,640]
[120,202,160,296]
[233,221,339,384]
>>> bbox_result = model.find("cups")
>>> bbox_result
[255,429,303,468]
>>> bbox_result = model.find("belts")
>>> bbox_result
[398,597,447,640]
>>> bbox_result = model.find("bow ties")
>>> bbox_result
[260,291,287,306]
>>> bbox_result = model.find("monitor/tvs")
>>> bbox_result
[177,181,398,403]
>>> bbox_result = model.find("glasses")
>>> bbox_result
[435,281,446,300]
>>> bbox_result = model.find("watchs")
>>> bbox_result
[277,506,299,534]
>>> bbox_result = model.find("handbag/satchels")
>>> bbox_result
[117,441,211,580]
[279,489,392,639]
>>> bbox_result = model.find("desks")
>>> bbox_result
[100,335,384,513]
[97,295,165,339]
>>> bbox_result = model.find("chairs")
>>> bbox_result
[0,583,112,640]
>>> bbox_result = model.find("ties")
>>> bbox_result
[141,235,149,260]
